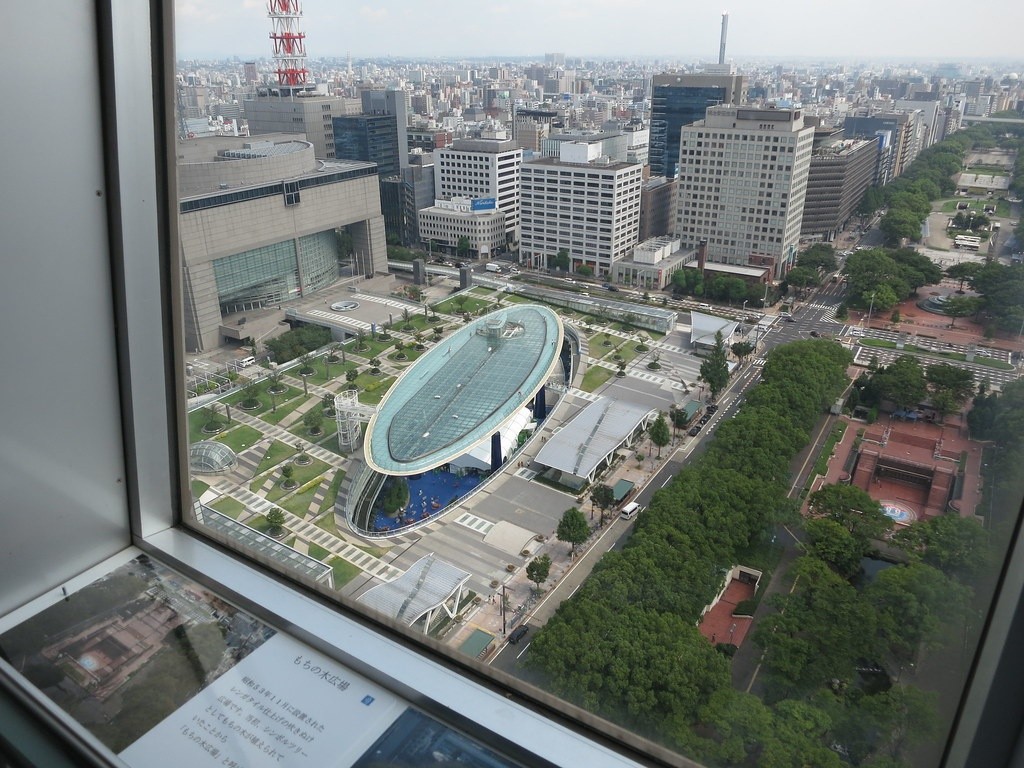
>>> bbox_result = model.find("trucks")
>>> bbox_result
[486,263,502,273]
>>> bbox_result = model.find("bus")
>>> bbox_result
[240,357,256,367]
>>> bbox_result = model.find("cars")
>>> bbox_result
[443,262,452,267]
[700,414,711,424]
[563,277,619,297]
[811,331,820,337]
[459,262,468,268]
[778,311,797,322]
[510,267,520,274]
[690,426,700,436]
[708,405,718,415]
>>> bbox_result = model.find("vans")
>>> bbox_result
[699,302,712,311]
[620,501,641,520]
[508,624,528,645]
